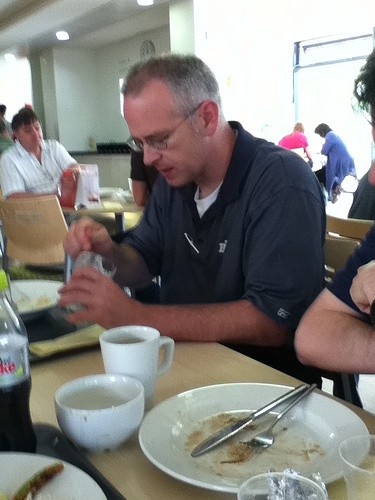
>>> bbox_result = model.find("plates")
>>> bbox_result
[1,451,108,500]
[10,279,66,320]
[138,383,371,493]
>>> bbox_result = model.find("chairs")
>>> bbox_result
[0,195,72,265]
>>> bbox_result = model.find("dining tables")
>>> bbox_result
[28,342,375,499]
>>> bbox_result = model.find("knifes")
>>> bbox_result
[191,383,309,458]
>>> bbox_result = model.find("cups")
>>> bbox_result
[99,325,175,398]
[237,472,327,500]
[339,435,375,500]
[66,250,118,312]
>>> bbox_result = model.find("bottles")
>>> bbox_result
[0,269,37,452]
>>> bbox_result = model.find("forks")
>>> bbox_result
[238,383,317,450]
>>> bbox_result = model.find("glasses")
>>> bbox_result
[127,99,207,153]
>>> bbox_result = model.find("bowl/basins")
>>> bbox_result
[100,187,135,203]
[53,373,145,450]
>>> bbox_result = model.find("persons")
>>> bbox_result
[58,53,331,398]
[0,102,355,244]
[295,50,375,374]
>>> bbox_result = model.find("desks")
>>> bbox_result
[62,188,145,235]
